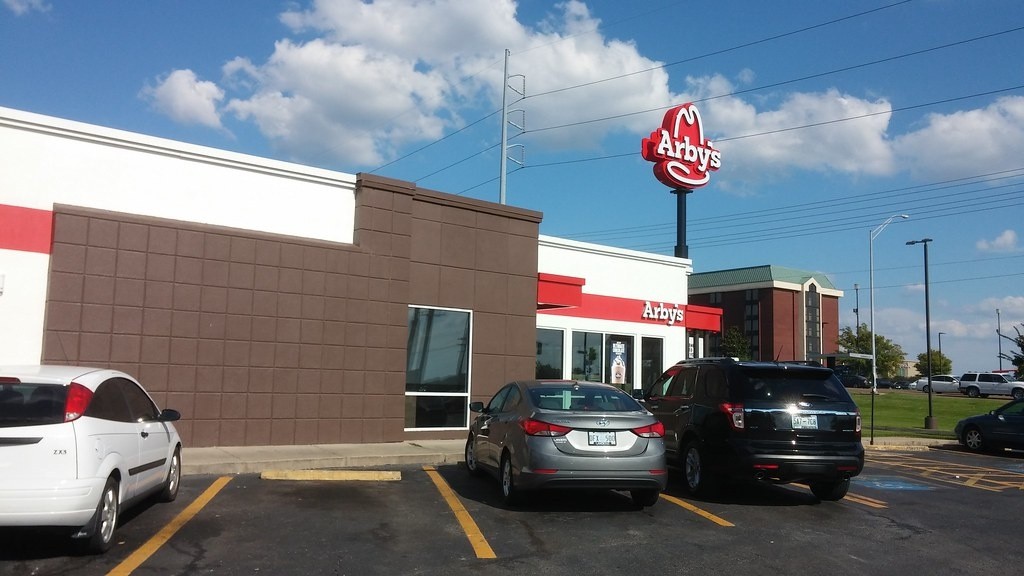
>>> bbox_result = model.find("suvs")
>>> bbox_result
[634,345,864,501]
[959,372,1024,400]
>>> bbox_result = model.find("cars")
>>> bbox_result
[0,365,181,554]
[876,377,909,390]
[465,378,668,507]
[833,365,871,388]
[916,373,963,394]
[954,396,1024,454]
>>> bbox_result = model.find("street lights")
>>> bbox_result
[996,309,1001,371]
[938,332,946,374]
[906,238,936,429]
[853,283,860,347]
[870,214,909,395]
